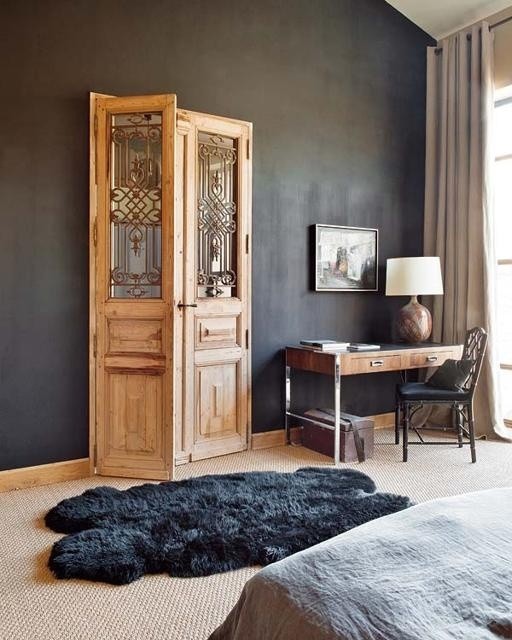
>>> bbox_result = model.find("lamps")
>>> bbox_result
[386,256,445,344]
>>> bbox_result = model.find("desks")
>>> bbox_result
[283,344,465,465]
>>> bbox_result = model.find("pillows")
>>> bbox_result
[425,359,478,392]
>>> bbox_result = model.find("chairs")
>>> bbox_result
[395,327,488,463]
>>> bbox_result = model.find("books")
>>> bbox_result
[345,342,381,351]
[298,339,350,349]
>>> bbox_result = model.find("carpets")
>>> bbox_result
[43,465,417,587]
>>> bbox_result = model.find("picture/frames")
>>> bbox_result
[313,223,380,293]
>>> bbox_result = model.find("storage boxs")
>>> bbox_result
[301,407,375,463]
